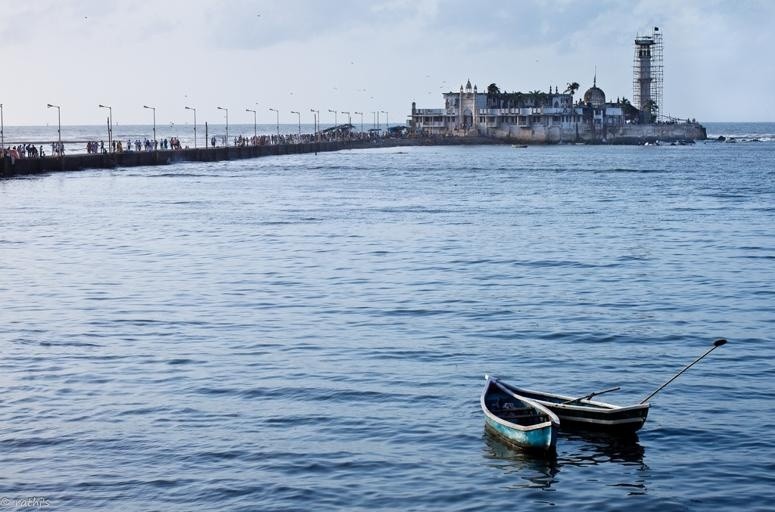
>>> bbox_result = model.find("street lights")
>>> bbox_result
[99,104,114,154]
[143,105,157,149]
[217,106,229,147]
[245,109,257,146]
[47,104,62,156]
[268,108,280,146]
[290,108,389,140]
[185,106,197,148]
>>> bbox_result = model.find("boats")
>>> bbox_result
[479,374,651,454]
[512,144,528,148]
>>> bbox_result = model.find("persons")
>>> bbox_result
[6,144,46,157]
[113,137,180,152]
[234,131,375,148]
[87,140,107,154]
[211,136,216,147]
[51,142,65,157]
